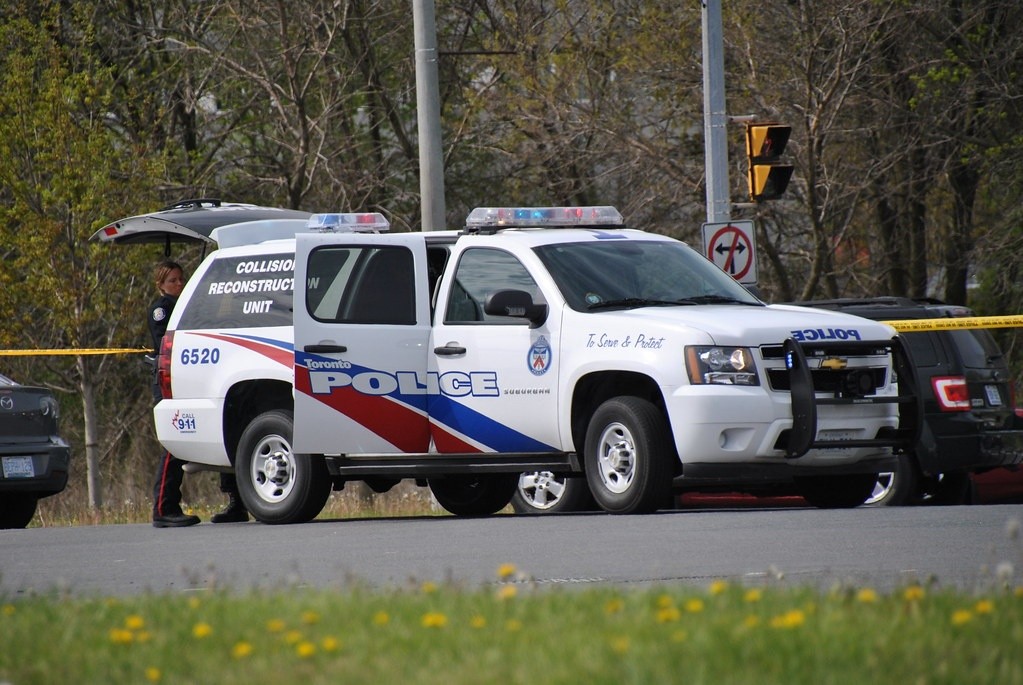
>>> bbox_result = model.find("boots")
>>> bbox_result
[211,493,249,523]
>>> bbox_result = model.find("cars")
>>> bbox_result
[84,199,923,523]
[0,374,72,529]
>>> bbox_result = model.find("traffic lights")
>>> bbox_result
[744,120,794,205]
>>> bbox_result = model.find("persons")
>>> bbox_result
[568,256,617,313]
[147,260,250,527]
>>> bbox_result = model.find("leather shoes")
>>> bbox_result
[179,512,200,524]
[153,512,194,527]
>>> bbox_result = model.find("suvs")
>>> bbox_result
[780,295,1023,511]
